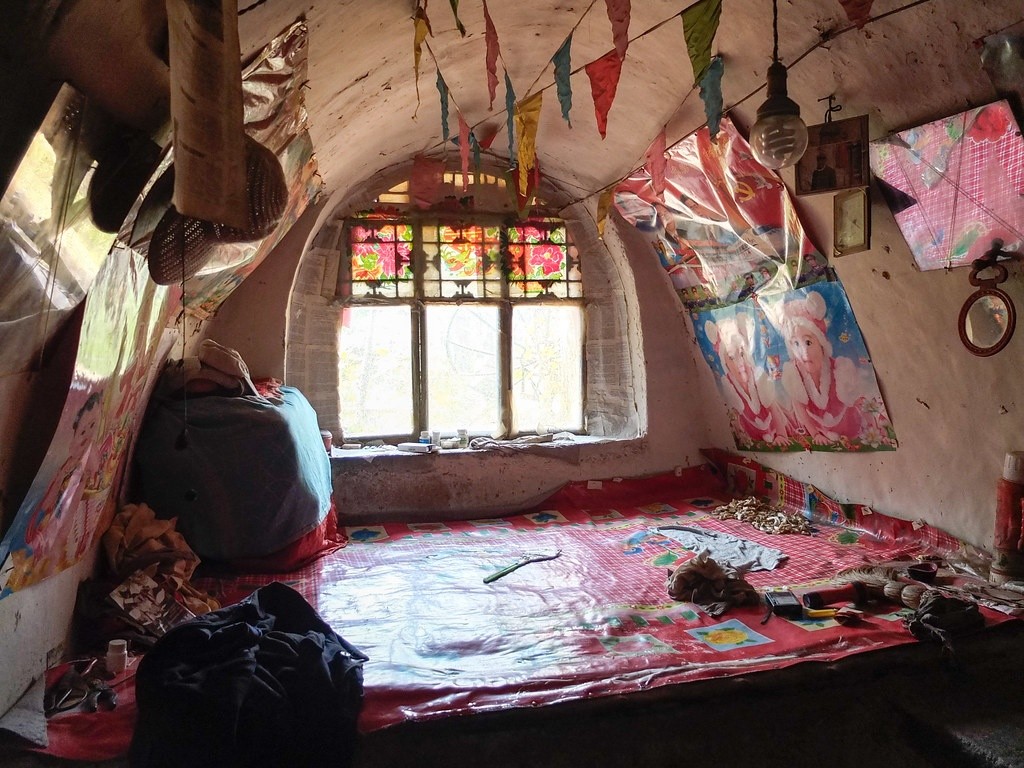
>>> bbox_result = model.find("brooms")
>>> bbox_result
[827,564,980,625]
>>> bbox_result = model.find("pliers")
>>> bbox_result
[87,678,118,712]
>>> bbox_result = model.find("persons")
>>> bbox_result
[741,272,759,294]
[704,312,800,448]
[803,254,827,276]
[701,285,717,304]
[790,260,807,283]
[691,287,704,306]
[679,194,726,240]
[652,201,689,241]
[681,288,695,307]
[759,267,775,288]
[727,279,747,298]
[778,292,873,442]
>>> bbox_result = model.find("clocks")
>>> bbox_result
[832,187,870,258]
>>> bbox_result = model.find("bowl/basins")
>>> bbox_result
[907,561,938,582]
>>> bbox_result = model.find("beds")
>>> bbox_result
[19,495,1024,768]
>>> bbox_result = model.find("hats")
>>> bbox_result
[766,291,834,361]
[149,133,289,286]
[704,312,756,376]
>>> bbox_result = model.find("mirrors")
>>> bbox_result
[958,265,1018,357]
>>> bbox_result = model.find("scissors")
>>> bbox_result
[43,658,98,712]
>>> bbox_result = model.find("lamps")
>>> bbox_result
[749,0,809,169]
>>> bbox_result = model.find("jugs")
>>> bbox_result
[987,451,1024,589]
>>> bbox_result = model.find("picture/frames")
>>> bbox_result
[795,114,869,196]
